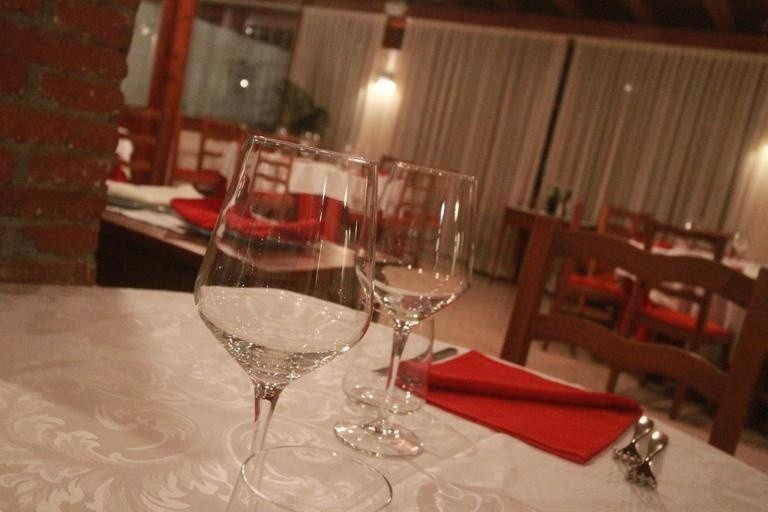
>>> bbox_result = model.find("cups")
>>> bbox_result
[218,446,392,512]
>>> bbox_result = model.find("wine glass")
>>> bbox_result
[733,230,750,269]
[195,134,379,507]
[332,161,477,460]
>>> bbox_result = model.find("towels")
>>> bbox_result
[395,350,643,464]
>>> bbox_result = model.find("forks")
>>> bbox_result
[611,412,656,466]
[630,429,671,491]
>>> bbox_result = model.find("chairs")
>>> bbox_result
[101,105,768,454]
